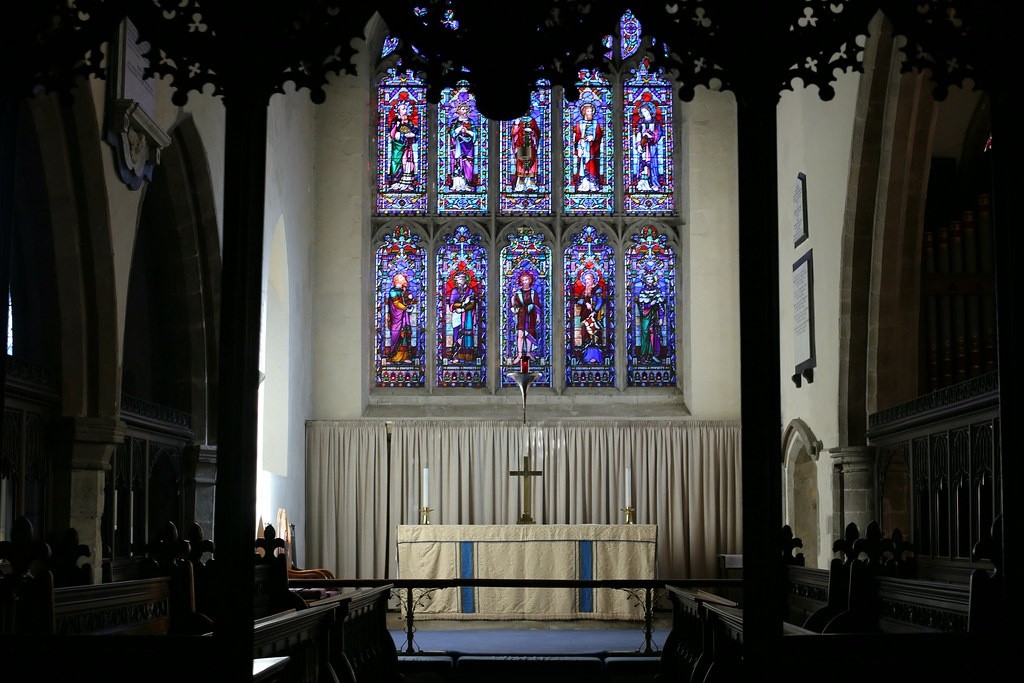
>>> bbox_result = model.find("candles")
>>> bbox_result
[625,467,631,508]
[423,468,429,508]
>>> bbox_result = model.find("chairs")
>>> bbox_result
[276,506,336,579]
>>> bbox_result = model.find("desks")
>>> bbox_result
[397,523,659,625]
